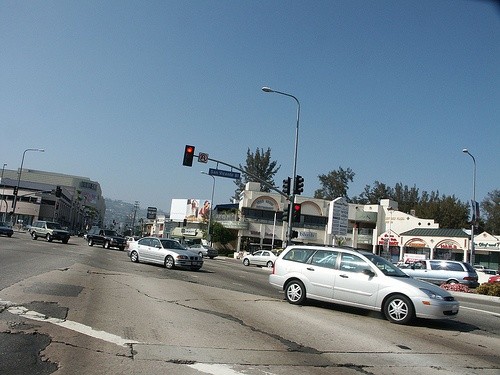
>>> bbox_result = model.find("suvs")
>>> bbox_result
[29,220,71,244]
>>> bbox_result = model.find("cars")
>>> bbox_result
[270,248,500,290]
[0,221,13,237]
[268,249,460,325]
[186,244,218,259]
[127,236,204,272]
[83,228,128,251]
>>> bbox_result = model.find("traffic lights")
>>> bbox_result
[282,203,290,223]
[13,186,18,195]
[292,203,301,223]
[183,145,195,166]
[55,186,61,197]
[282,177,290,195]
[295,175,304,193]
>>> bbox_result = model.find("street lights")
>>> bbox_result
[12,148,46,214]
[261,86,300,245]
[200,171,215,234]
[462,148,476,267]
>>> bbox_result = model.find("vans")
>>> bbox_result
[240,249,282,268]
